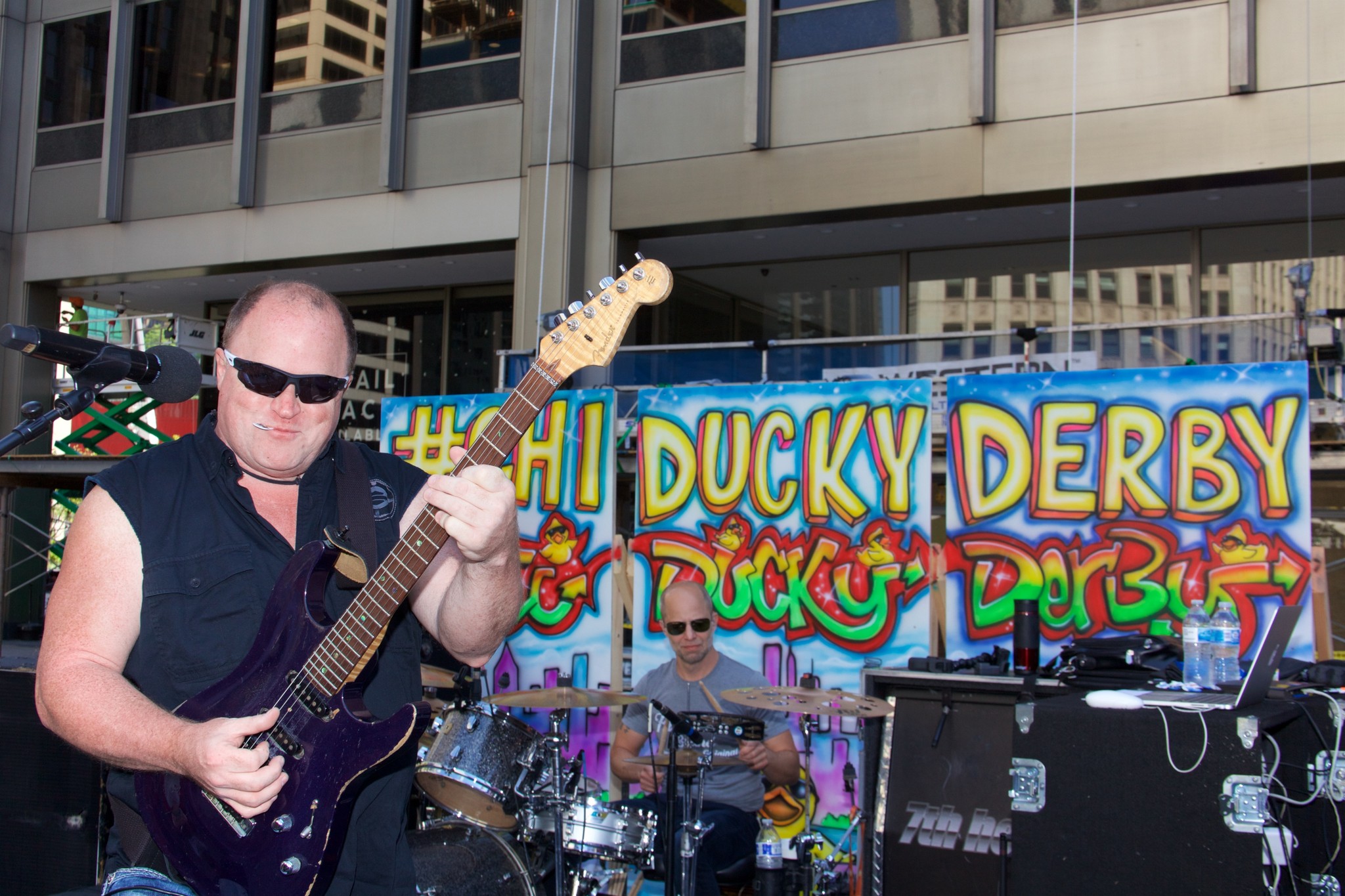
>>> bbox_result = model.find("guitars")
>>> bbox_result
[132,252,676,896]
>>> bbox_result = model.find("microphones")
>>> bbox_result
[932,700,954,748]
[650,699,703,745]
[1,324,202,403]
[998,832,1007,896]
[564,749,584,793]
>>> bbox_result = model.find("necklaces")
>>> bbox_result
[239,466,302,485]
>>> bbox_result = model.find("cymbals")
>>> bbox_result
[419,663,459,688]
[720,687,897,717]
[483,686,652,709]
[619,747,752,772]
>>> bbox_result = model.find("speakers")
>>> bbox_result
[0,637,110,895]
[870,689,1017,896]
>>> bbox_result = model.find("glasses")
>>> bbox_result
[223,349,351,405]
[666,617,712,635]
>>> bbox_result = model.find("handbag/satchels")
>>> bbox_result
[1060,634,1184,670]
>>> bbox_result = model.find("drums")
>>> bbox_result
[520,796,660,867]
[415,811,557,896]
[677,710,766,741]
[412,700,546,835]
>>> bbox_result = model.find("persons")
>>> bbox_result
[33,280,523,895]
[609,580,800,896]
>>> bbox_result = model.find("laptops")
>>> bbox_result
[1134,605,1304,710]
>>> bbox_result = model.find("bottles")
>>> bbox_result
[1181,598,1213,688]
[757,819,783,869]
[1208,602,1240,684]
